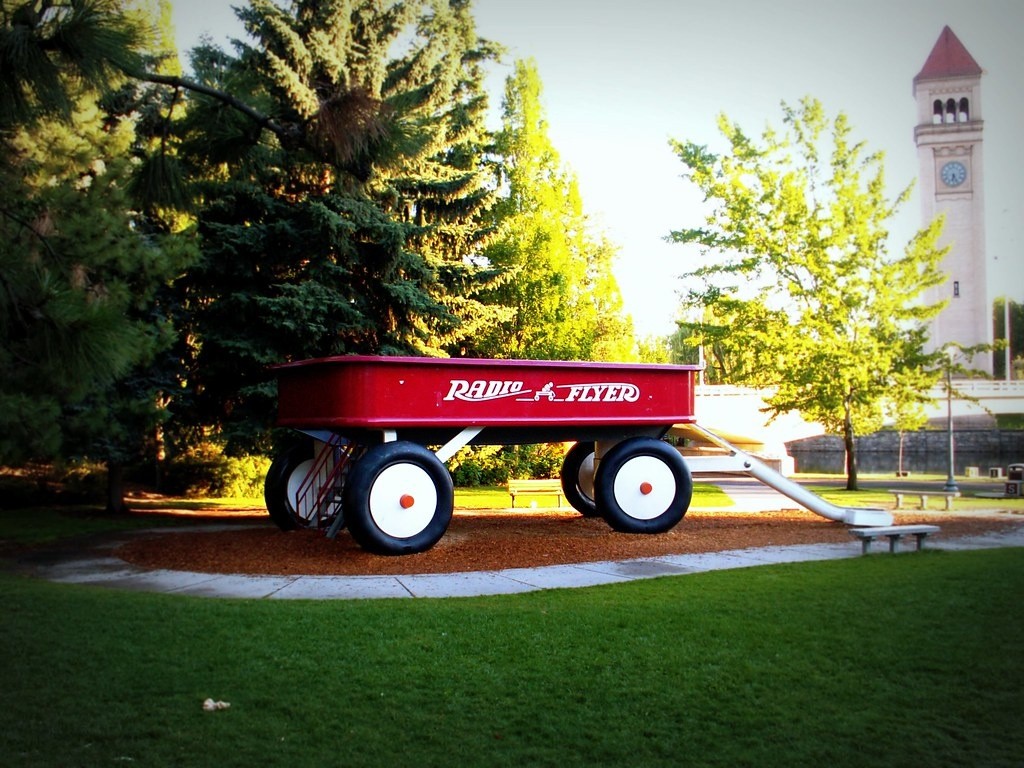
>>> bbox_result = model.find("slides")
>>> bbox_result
[728,448,896,528]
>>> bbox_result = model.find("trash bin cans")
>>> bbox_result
[1007,462,1024,496]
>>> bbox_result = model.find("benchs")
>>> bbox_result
[888,490,960,510]
[849,524,941,556]
[508,479,563,507]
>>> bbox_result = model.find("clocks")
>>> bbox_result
[941,161,966,187]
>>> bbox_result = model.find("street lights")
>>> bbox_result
[942,345,959,492]
